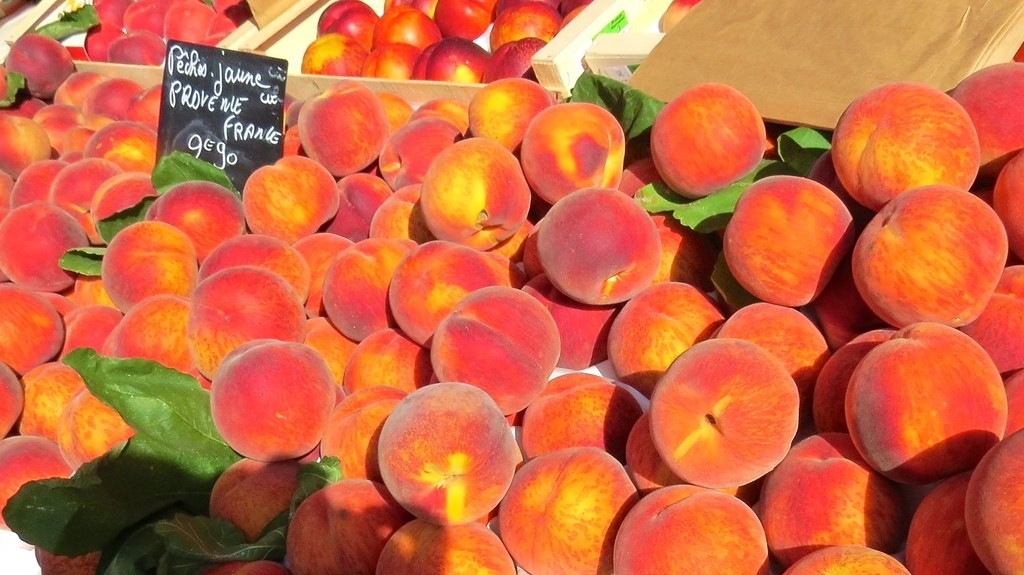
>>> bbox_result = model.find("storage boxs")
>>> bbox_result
[4,0,257,89]
[585,0,1024,121]
[241,0,639,109]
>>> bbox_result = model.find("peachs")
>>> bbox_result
[0,0,1024,575]
